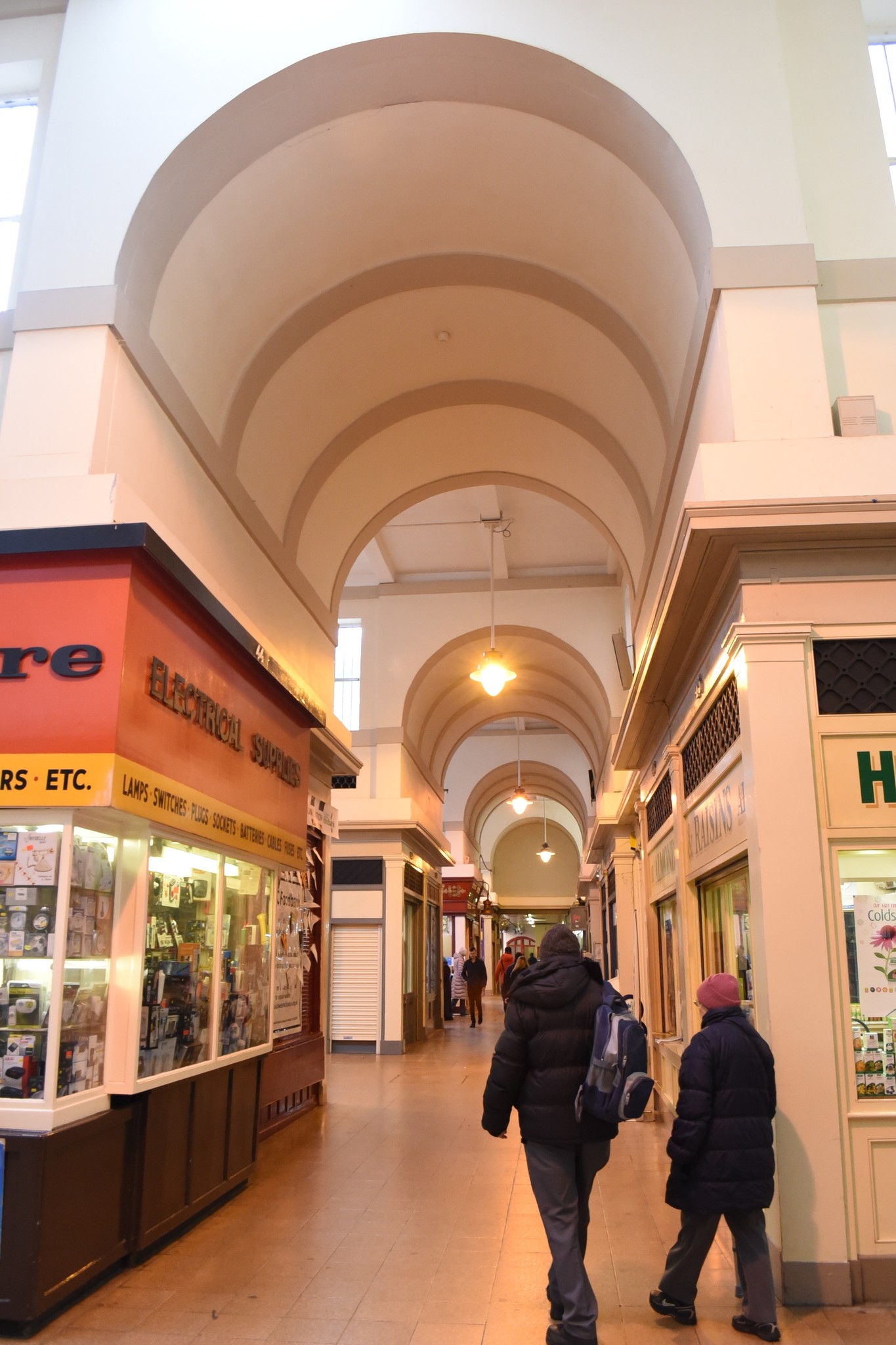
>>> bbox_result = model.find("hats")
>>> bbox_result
[697,973,741,1008]
[540,924,580,960]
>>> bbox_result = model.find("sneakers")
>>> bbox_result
[732,1313,780,1340]
[649,1289,696,1324]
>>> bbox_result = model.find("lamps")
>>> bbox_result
[536,797,556,862]
[507,717,533,815]
[478,881,492,915]
[469,520,519,695]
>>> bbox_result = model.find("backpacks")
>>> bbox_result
[569,980,655,1125]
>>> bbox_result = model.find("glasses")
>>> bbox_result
[694,1000,701,1007]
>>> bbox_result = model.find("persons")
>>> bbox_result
[528,952,537,966]
[509,956,529,988]
[494,946,514,1012]
[650,972,781,1342]
[481,923,610,1345]
[461,947,487,1027]
[443,957,453,1021]
[451,947,471,1017]
[504,952,522,993]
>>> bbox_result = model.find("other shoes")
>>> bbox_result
[546,1285,564,1320]
[478,1016,482,1025]
[470,1021,475,1027]
[546,1322,598,1345]
[463,1014,470,1017]
